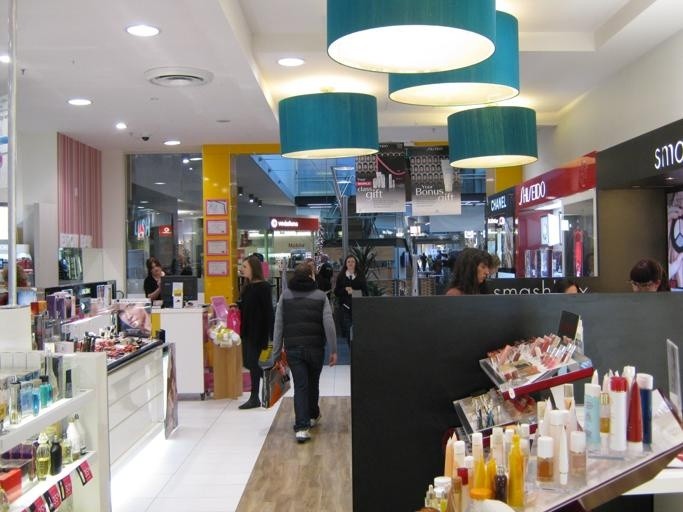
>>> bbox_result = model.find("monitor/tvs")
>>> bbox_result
[43,279,119,301]
[160,274,199,302]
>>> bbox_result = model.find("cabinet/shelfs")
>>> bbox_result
[37,280,116,309]
[152,301,212,401]
[448,388,679,511]
[60,311,166,473]
[0,350,111,511]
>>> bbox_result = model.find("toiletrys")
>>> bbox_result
[558,426,568,485]
[424,364,655,511]
[355,170,396,189]
[0,369,88,511]
[626,376,644,452]
[440,159,454,193]
[31,283,112,351]
[542,396,552,435]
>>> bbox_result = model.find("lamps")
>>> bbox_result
[388,10,521,106]
[277,92,380,160]
[446,106,538,168]
[327,0,497,76]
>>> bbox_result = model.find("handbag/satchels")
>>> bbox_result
[262,360,290,409]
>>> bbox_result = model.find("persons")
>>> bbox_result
[490,256,501,274]
[400,248,450,284]
[228,255,273,410]
[144,256,173,301]
[249,252,333,302]
[668,205,682,283]
[552,280,577,294]
[1,264,27,306]
[630,257,672,293]
[335,254,369,350]
[269,262,338,443]
[118,303,151,336]
[445,248,494,296]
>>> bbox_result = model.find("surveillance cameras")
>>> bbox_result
[141,132,150,142]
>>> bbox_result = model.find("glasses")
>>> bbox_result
[630,280,655,290]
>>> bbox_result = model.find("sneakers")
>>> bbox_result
[310,413,321,427]
[238,396,260,409]
[296,429,311,441]
